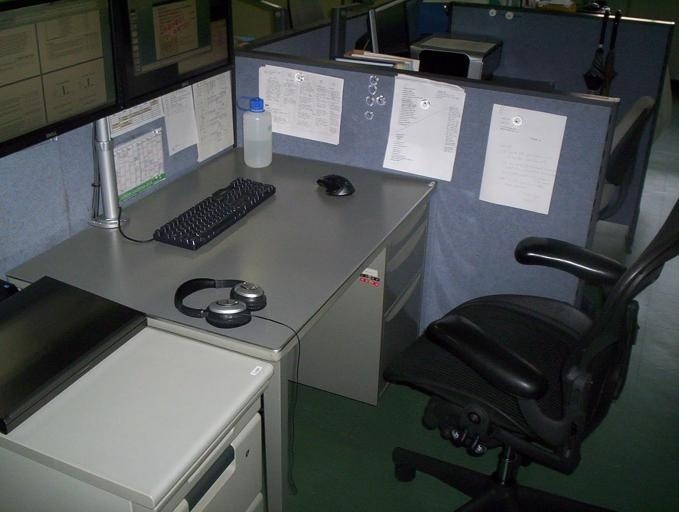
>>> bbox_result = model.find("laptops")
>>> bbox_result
[0,275,148,435]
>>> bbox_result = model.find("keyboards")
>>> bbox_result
[153,178,275,251]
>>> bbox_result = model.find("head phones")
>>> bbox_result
[174,278,267,328]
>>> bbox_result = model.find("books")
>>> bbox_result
[480,1,578,14]
[337,49,421,73]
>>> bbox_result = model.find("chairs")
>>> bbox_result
[394,199,679,512]
[597,96,657,218]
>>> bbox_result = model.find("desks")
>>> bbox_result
[7,150,438,511]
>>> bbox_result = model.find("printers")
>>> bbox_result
[409,32,502,80]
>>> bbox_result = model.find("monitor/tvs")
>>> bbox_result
[0,0,125,157]
[108,0,235,110]
[368,0,410,59]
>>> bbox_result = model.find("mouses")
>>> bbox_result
[317,175,355,196]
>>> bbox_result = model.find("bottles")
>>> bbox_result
[243,95,274,170]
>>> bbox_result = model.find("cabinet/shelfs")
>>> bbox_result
[0,328,273,511]
